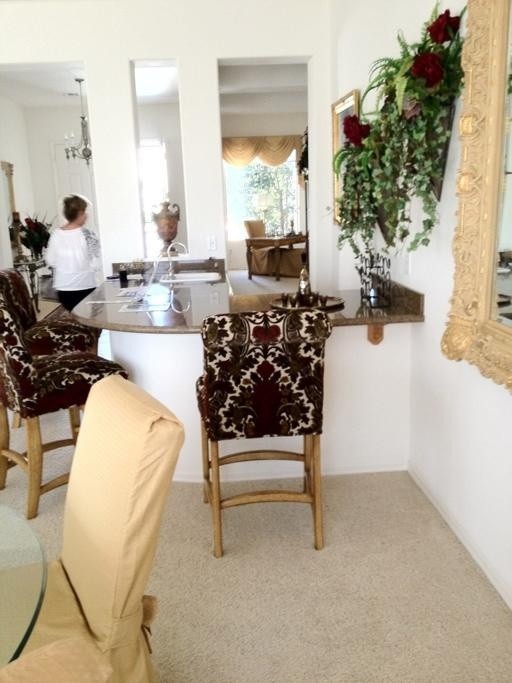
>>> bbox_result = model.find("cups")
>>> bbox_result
[282,291,328,307]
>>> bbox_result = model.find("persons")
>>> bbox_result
[44,194,103,312]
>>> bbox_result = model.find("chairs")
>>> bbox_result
[244,220,275,277]
[2,373,186,682]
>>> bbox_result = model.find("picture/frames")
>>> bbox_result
[440,0,512,391]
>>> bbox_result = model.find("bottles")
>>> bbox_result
[298,268,311,303]
[119,265,128,288]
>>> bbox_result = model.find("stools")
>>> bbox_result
[195,309,334,557]
[1,270,100,429]
[1,301,128,520]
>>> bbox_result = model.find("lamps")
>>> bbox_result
[64,78,92,167]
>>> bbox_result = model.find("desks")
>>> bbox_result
[13,258,46,313]
[245,236,306,281]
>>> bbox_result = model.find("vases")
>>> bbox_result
[9,212,24,248]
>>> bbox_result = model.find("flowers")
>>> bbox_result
[332,1,469,258]
[19,210,59,249]
